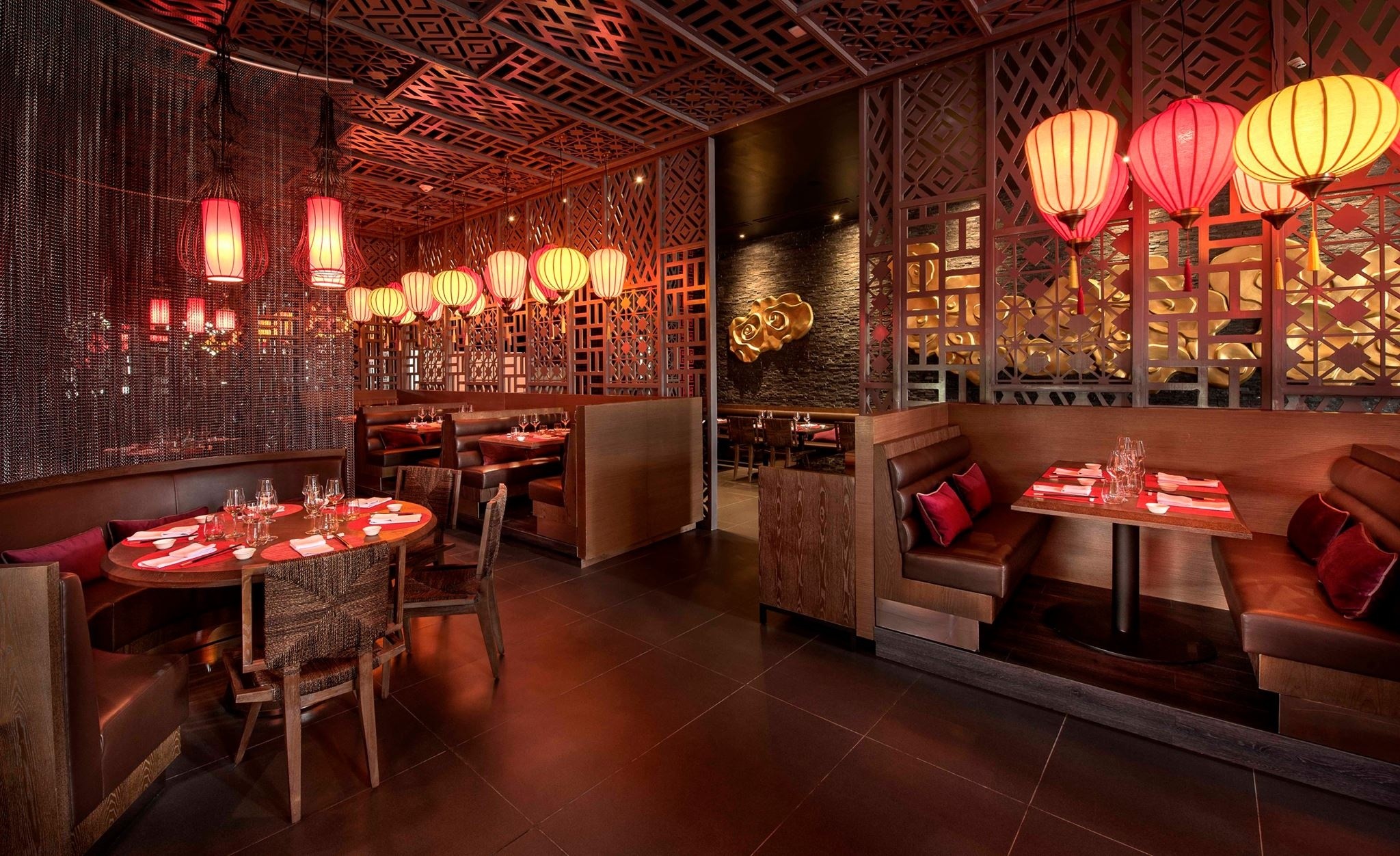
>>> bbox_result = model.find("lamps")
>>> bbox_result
[289,0,364,293]
[178,0,260,298]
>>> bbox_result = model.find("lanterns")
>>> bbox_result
[178,25,269,285]
[290,82,368,292]
[89,298,237,354]
[1023,69,1400,314]
[347,245,629,335]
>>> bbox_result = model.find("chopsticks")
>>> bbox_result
[332,532,353,549]
[1152,472,1220,482]
[1049,475,1106,479]
[1033,492,1099,499]
[180,544,242,566]
[369,512,422,515]
[129,534,198,544]
[1144,490,1215,501]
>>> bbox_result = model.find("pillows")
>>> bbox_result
[1284,491,1351,560]
[1,523,109,581]
[915,478,976,546]
[1314,519,1397,621]
[107,504,209,549]
[952,460,995,510]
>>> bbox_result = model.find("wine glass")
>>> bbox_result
[560,411,570,432]
[223,474,345,541]
[1105,436,1146,502]
[519,412,541,437]
[417,406,437,426]
[794,410,811,429]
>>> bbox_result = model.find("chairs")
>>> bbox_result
[401,477,517,687]
[226,550,389,823]
[725,414,856,482]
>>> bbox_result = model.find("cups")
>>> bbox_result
[342,498,360,521]
[410,417,417,426]
[1101,477,1125,505]
[246,518,269,547]
[318,511,339,536]
[511,427,520,441]
[540,425,547,434]
[553,423,562,435]
[204,513,226,541]
[458,404,475,413]
[574,410,577,422]
[436,415,443,426]
[758,410,772,419]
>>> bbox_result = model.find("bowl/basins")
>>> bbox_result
[1077,478,1096,486]
[1158,482,1179,491]
[153,539,176,550]
[362,526,382,536]
[232,547,256,560]
[1085,463,1102,470]
[1145,503,1170,514]
[387,503,403,512]
[194,514,210,524]
[516,437,525,441]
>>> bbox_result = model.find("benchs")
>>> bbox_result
[876,423,1054,651]
[1210,444,1400,765]
[718,402,864,449]
[0,434,361,856]
[299,387,579,544]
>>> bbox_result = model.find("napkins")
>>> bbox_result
[1052,463,1104,478]
[348,496,393,508]
[1154,470,1220,488]
[286,535,335,556]
[369,513,422,524]
[246,501,288,515]
[1033,481,1094,496]
[1156,490,1232,513]
[138,540,217,570]
[125,523,199,542]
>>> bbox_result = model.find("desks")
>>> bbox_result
[384,423,442,434]
[477,424,572,450]
[1010,457,1255,665]
[95,483,438,719]
[718,413,834,472]
